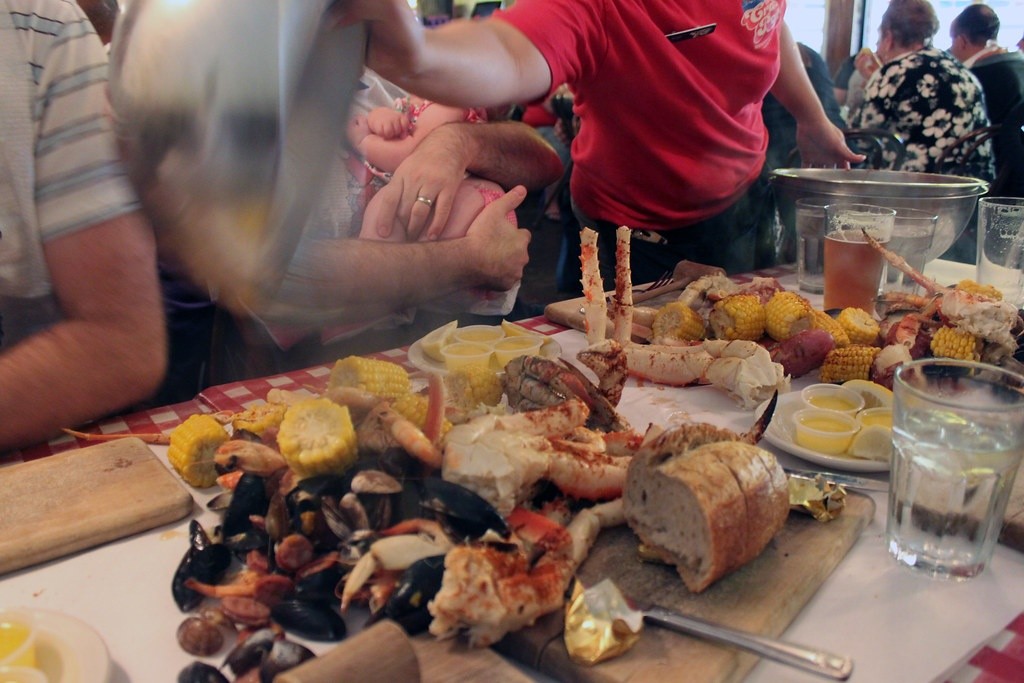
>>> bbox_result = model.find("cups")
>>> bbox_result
[795,197,841,293]
[877,205,938,296]
[975,197,1024,310]
[823,202,896,320]
[886,359,1024,581]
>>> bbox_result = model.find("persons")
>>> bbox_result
[0,0,1024,453]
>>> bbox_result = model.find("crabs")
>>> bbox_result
[186,221,1020,651]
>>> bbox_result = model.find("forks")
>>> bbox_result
[579,271,675,313]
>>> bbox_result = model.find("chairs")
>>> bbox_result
[786,129,904,170]
[935,124,1024,257]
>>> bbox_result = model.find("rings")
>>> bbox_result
[417,197,433,207]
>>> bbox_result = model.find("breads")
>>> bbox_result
[624,421,791,594]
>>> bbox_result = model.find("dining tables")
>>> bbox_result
[0,259,1024,683]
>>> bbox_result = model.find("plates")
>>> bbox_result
[0,605,112,683]
[754,391,889,474]
[406,328,562,373]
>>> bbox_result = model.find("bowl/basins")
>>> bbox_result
[799,383,865,419]
[792,407,860,456]
[439,342,494,372]
[767,166,989,266]
[454,324,506,345]
[856,407,890,430]
[494,334,544,366]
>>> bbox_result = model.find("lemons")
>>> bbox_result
[420,319,458,361]
[502,320,552,346]
[839,378,895,410]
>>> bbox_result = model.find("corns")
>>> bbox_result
[167,354,503,488]
[652,279,1002,383]
